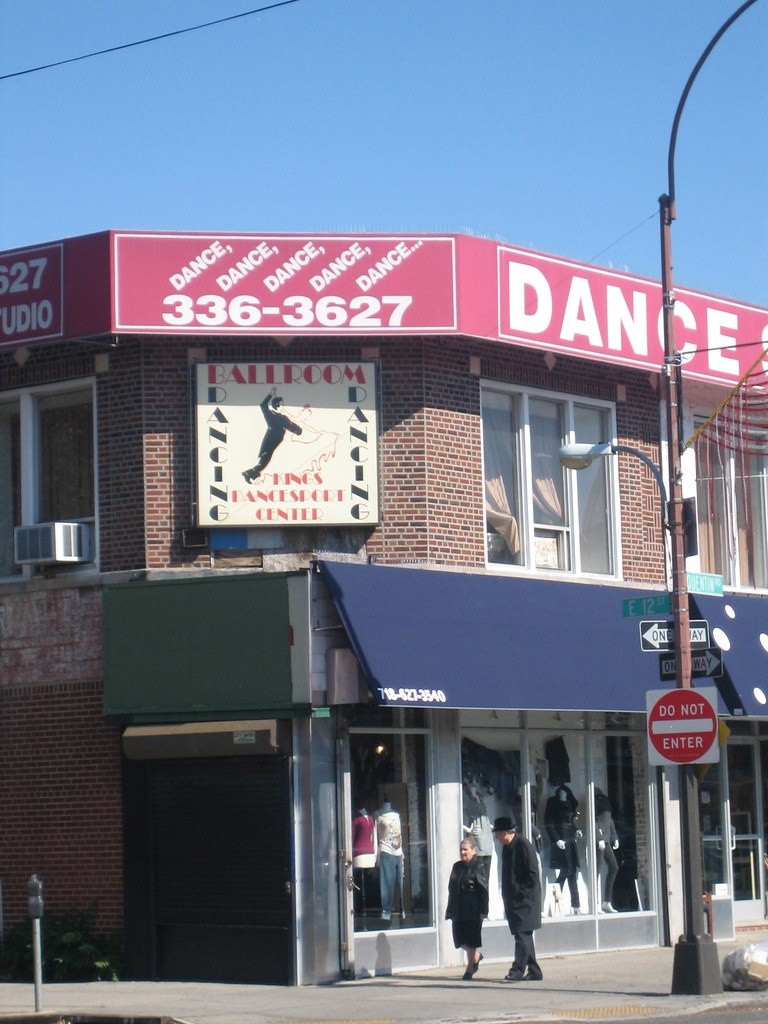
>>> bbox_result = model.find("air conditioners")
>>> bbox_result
[13,521,90,565]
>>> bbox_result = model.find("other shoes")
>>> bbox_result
[463,972,473,979]
[473,953,484,973]
[523,973,542,980]
[505,974,521,980]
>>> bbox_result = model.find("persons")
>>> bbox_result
[373,802,406,920]
[462,785,494,883]
[492,817,542,983]
[544,786,583,915]
[512,786,543,883]
[351,808,378,868]
[444,838,488,980]
[594,786,619,913]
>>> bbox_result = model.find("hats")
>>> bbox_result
[493,817,515,831]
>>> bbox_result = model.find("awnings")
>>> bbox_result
[690,594,768,717]
[311,559,734,714]
